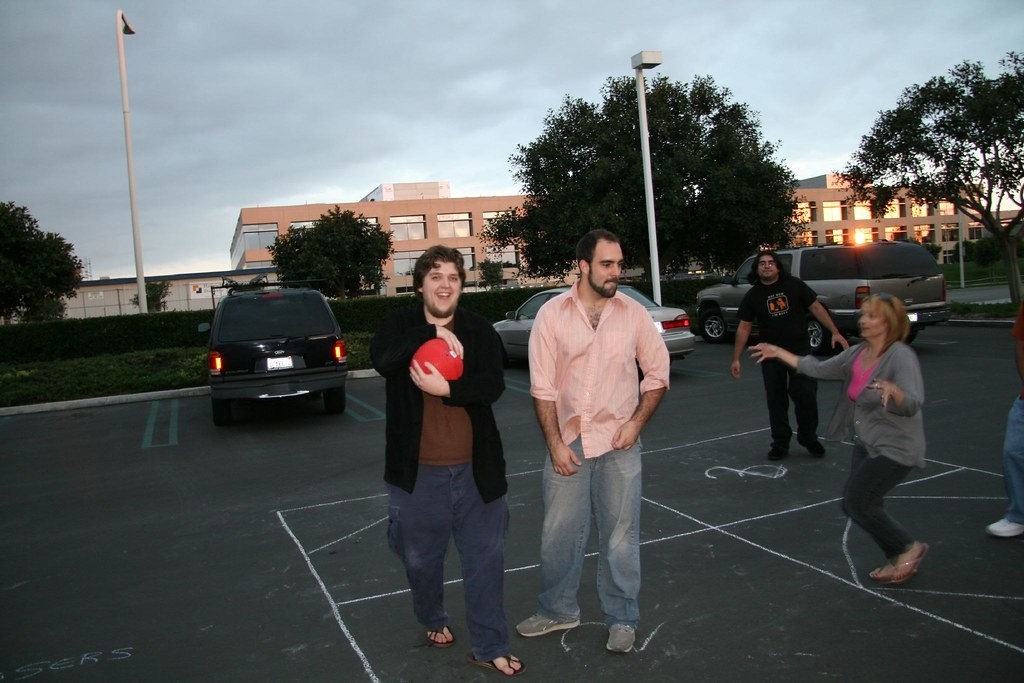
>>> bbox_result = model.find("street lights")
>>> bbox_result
[114,9,149,314]
[631,51,662,308]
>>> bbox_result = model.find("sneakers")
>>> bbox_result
[515,612,580,637]
[605,623,636,652]
[985,517,1024,537]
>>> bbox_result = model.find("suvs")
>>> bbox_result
[692,240,951,352]
[198,281,349,425]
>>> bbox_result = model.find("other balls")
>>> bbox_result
[411,339,464,380]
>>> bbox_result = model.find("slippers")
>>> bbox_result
[875,542,929,582]
[466,651,527,676]
[426,625,456,648]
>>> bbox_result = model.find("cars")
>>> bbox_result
[492,285,695,380]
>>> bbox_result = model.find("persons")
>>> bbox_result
[748,292,928,584]
[516,228,671,654]
[986,300,1024,537]
[368,245,526,677]
[729,249,850,461]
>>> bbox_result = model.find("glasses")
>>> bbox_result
[758,262,776,267]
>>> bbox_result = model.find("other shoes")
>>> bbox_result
[768,445,789,460]
[797,437,825,456]
